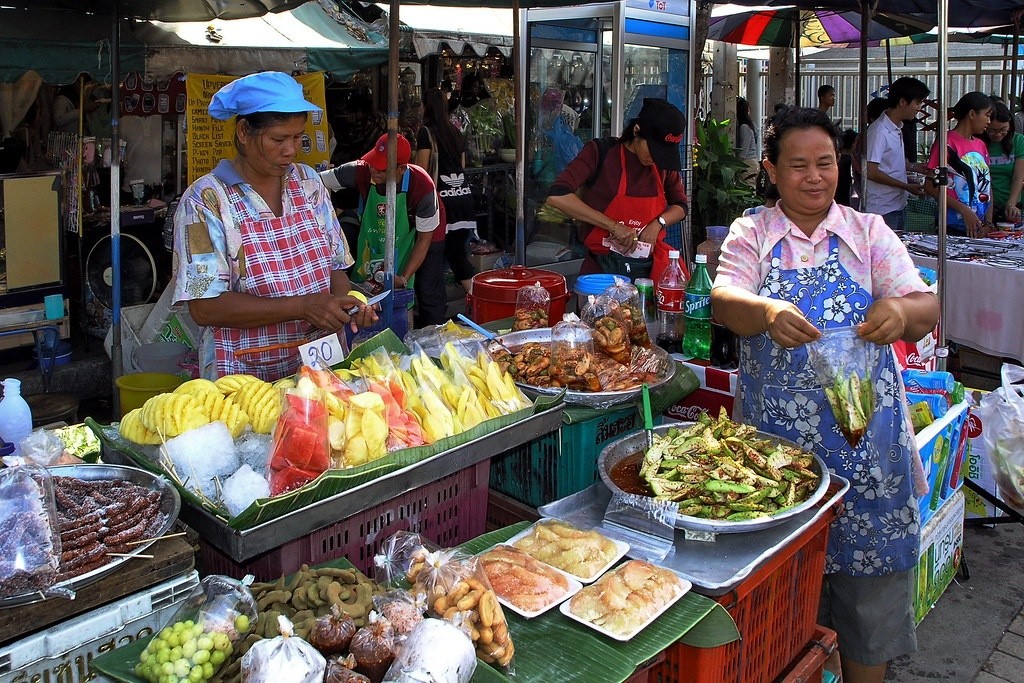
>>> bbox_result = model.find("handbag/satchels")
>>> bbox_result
[582,244,653,284]
[980,362,1024,516]
[421,125,438,190]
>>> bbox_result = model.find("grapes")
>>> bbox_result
[135,615,247,683]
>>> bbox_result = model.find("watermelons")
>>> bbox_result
[268,365,422,497]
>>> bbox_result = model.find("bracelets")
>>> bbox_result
[400,274,408,286]
[910,163,915,171]
[607,221,624,237]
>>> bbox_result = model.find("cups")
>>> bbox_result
[633,277,658,345]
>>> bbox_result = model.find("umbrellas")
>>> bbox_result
[706,4,1012,106]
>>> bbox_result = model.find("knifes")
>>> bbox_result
[304,289,392,335]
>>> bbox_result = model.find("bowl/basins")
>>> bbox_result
[477,326,677,407]
[501,149,516,162]
[597,421,831,533]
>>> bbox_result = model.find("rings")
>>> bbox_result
[633,237,638,241]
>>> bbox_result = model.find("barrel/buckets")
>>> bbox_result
[345,287,414,354]
[573,273,632,319]
[133,342,188,376]
[115,372,183,421]
[465,265,572,329]
[34,351,73,369]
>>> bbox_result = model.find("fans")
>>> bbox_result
[87,233,157,312]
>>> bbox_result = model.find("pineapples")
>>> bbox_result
[118,373,389,468]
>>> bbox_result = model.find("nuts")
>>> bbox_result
[406,549,515,663]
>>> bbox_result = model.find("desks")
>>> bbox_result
[663,351,970,628]
[905,199,938,233]
[464,163,530,248]
[908,253,1024,384]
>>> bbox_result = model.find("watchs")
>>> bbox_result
[656,216,666,229]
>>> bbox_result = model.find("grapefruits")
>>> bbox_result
[477,521,684,637]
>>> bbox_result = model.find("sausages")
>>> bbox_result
[0,474,165,599]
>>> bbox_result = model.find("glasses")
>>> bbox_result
[986,129,1009,135]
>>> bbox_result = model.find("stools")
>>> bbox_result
[23,393,80,425]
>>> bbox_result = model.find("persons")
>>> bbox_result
[737,77,1024,234]
[315,76,616,336]
[710,108,945,683]
[52,77,102,137]
[547,98,690,277]
[171,70,378,388]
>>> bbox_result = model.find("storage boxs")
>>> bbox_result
[0,390,844,683]
[345,286,415,352]
[472,251,505,272]
[104,303,198,376]
[534,221,592,260]
[0,299,70,350]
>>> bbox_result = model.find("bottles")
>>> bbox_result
[896,366,965,435]
[655,251,688,354]
[709,306,740,370]
[681,254,714,362]
[929,406,970,512]
[0,377,33,459]
[697,226,729,283]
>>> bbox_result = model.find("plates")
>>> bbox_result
[466,517,693,643]
[1,463,185,609]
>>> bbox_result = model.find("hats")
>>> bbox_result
[361,132,411,171]
[207,71,323,121]
[638,98,686,172]
